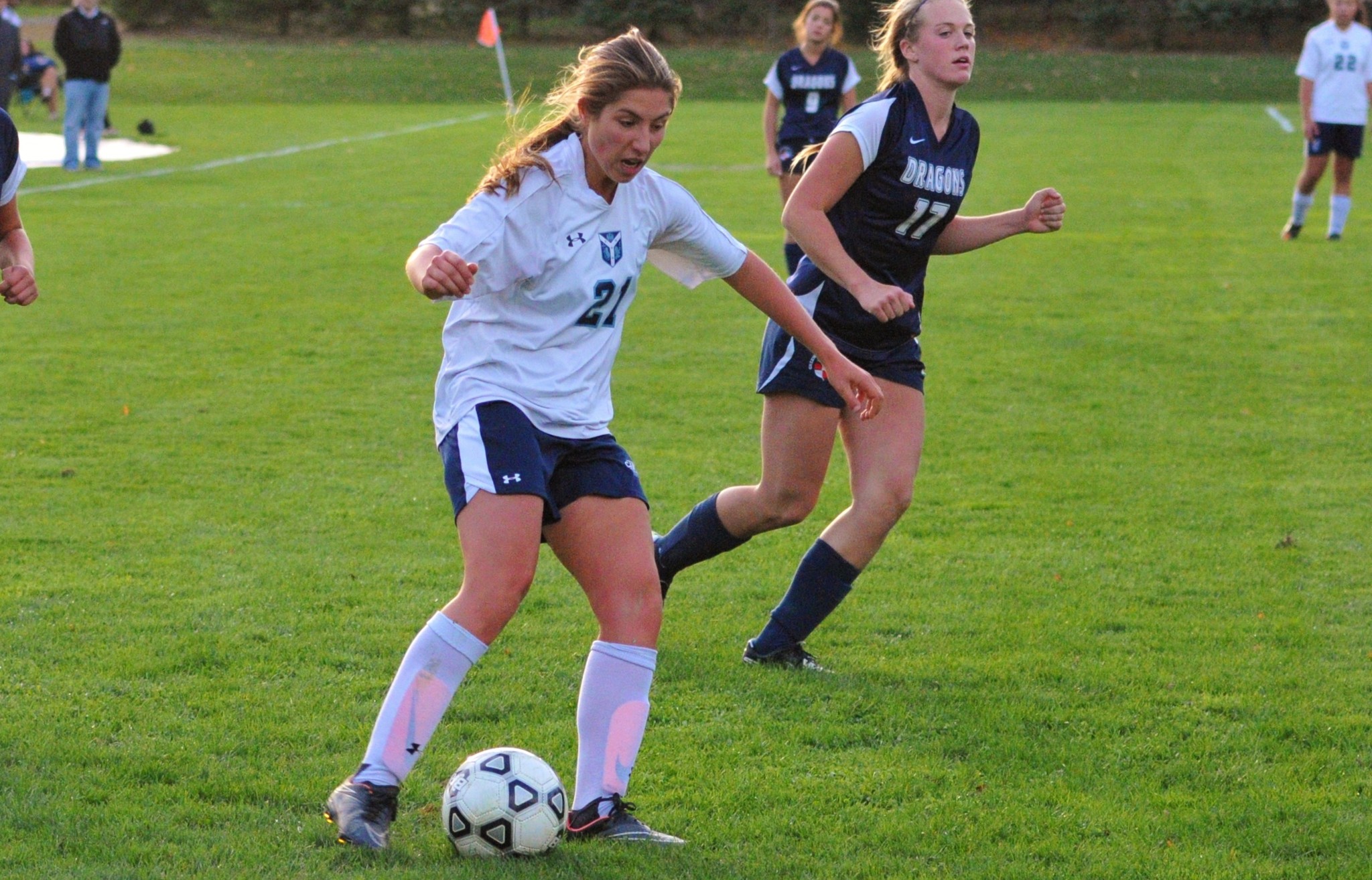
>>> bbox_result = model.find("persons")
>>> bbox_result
[319,21,889,856]
[0,0,30,114]
[1279,0,1371,242]
[0,105,39,307]
[762,0,862,280]
[642,0,1065,676]
[14,37,63,119]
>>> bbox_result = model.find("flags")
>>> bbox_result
[476,10,498,50]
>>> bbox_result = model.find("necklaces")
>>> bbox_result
[53,0,124,172]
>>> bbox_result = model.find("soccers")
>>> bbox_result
[439,747,569,859]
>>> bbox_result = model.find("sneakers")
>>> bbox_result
[321,773,399,859]
[742,638,833,673]
[1324,233,1340,240]
[1281,217,1304,240]
[561,810,689,846]
[651,530,672,608]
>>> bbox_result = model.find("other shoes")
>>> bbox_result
[83,160,102,171]
[66,160,77,171]
